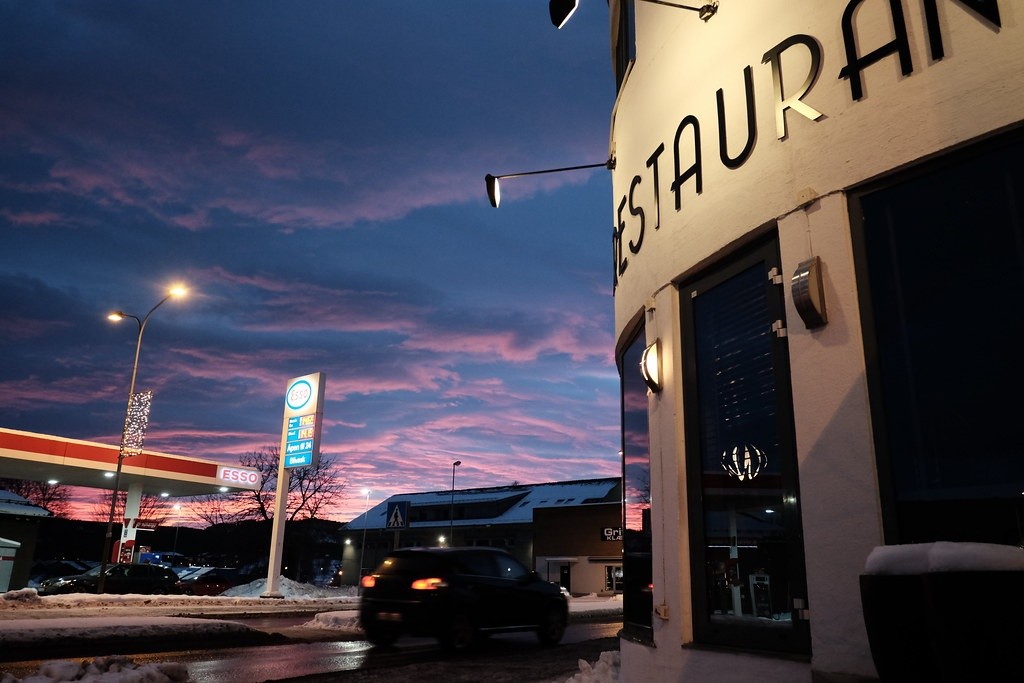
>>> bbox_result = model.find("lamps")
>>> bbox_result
[485,157,616,210]
[640,337,663,392]
[549,0,718,29]
[788,257,829,329]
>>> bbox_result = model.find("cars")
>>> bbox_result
[28,547,379,597]
[358,546,571,654]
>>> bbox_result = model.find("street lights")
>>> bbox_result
[95,282,193,596]
[450,461,464,546]
[357,487,372,593]
